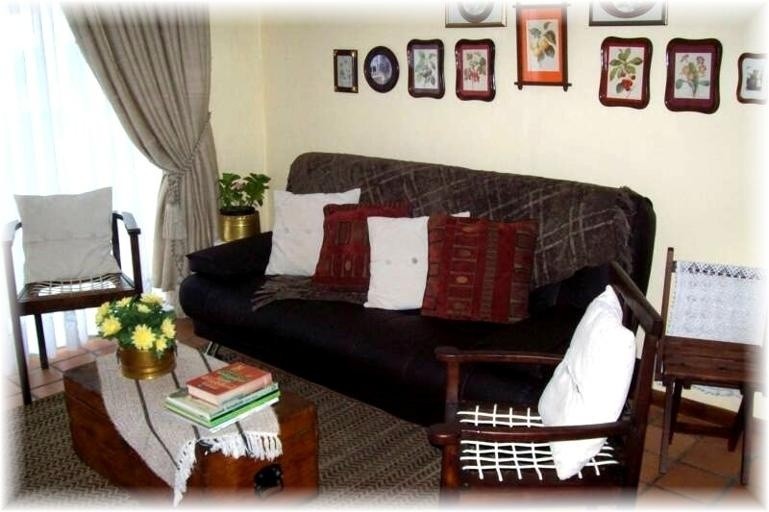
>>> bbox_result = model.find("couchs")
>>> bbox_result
[179,152,657,422]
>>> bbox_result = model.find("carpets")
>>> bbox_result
[8,342,442,507]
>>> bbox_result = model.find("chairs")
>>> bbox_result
[3,210,143,402]
[657,247,767,487]
[429,262,663,506]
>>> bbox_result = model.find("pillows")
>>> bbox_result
[17,188,121,282]
[424,214,534,323]
[313,203,413,294]
[538,286,638,481]
[265,190,362,276]
[366,212,474,310]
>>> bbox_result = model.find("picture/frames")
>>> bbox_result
[597,35,654,110]
[511,2,573,92]
[445,2,505,24]
[406,37,445,99]
[738,52,767,103]
[334,48,359,92]
[664,38,721,114]
[363,48,400,92]
[588,2,666,24]
[455,38,498,101]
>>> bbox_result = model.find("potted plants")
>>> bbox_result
[217,170,269,243]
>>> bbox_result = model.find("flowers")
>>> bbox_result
[97,295,177,361]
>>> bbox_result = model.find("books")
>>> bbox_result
[164,361,282,432]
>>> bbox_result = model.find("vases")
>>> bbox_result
[120,345,177,379]
